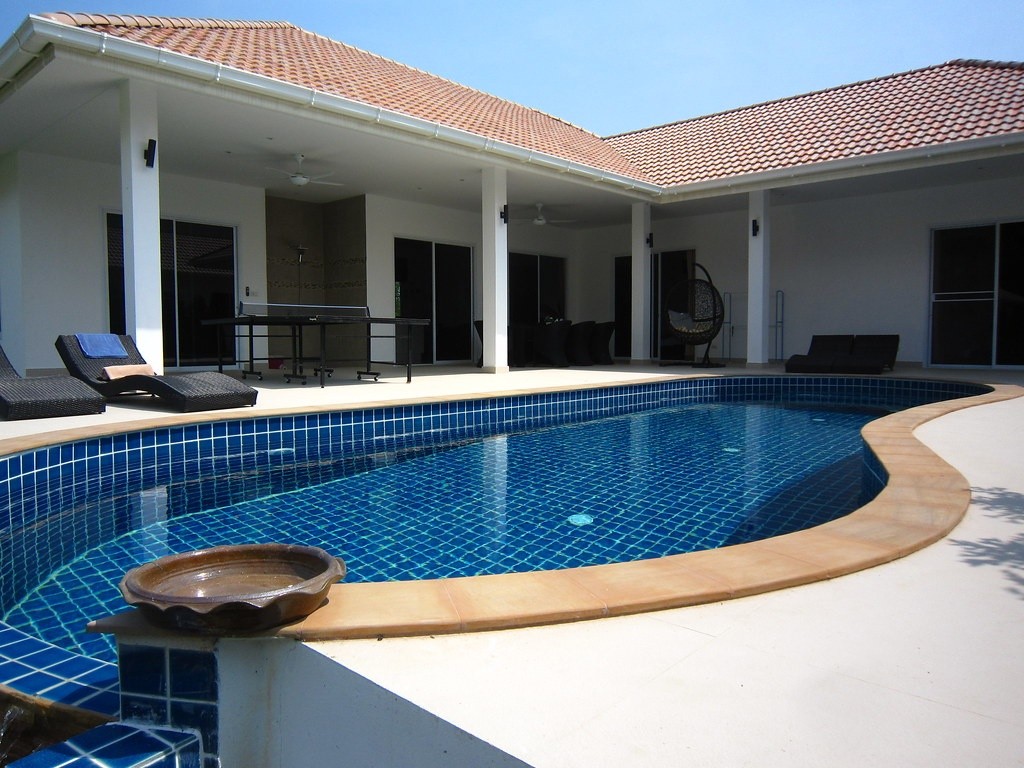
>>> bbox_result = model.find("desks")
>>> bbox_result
[208,317,430,383]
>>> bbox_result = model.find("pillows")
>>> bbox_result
[670,310,695,330]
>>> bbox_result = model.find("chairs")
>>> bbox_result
[0,346,104,419]
[831,334,900,374]
[54,333,258,413]
[473,319,616,367]
[784,335,855,373]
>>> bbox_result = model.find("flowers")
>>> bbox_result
[541,316,564,327]
